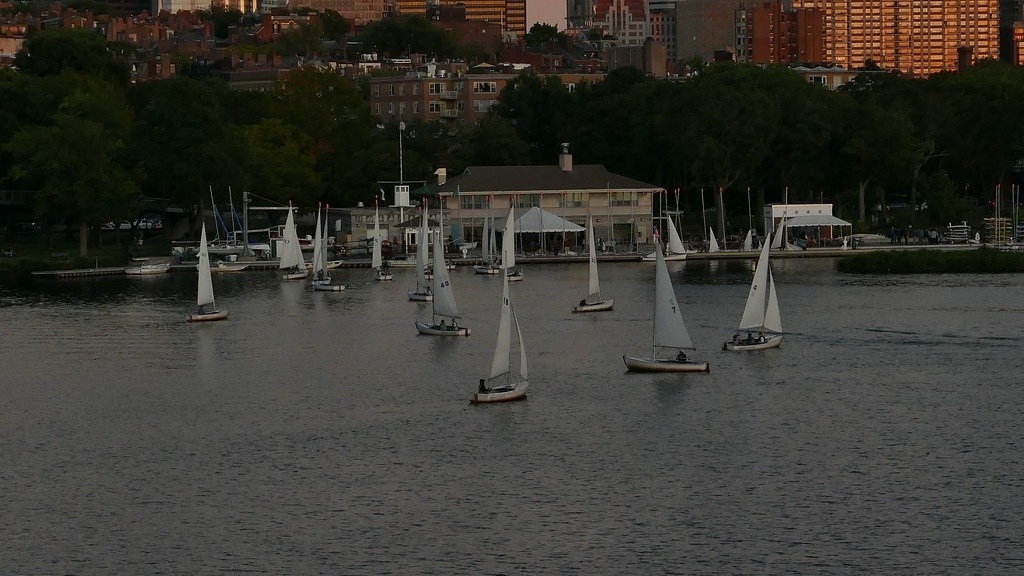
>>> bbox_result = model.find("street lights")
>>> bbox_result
[642,216,686,260]
[570,212,615,311]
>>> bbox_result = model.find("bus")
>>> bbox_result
[171,241,227,256]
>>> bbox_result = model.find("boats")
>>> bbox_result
[124,258,171,275]
[299,260,344,270]
[985,243,1019,250]
[945,224,971,241]
[196,260,249,272]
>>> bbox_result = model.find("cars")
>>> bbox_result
[103,219,162,230]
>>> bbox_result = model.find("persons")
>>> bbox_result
[377,266,381,274]
[564,239,570,254]
[294,265,299,274]
[467,229,470,243]
[451,319,458,330]
[746,331,765,344]
[318,268,324,281]
[752,226,756,239]
[423,286,432,295]
[449,235,454,245]
[581,238,585,249]
[580,298,587,306]
[478,379,490,394]
[440,319,446,330]
[890,227,939,245]
[800,231,808,240]
[393,236,398,243]
[676,350,687,361]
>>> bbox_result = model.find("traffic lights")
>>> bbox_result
[992,202,995,206]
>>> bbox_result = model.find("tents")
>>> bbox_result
[489,206,587,253]
[776,216,852,248]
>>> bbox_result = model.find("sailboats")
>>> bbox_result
[771,187,802,251]
[623,236,711,374]
[473,251,530,403]
[185,222,229,322]
[278,200,346,291]
[408,197,472,337]
[445,185,477,252]
[372,197,393,281]
[190,185,244,255]
[473,203,522,282]
[722,230,784,352]
[666,188,763,254]
